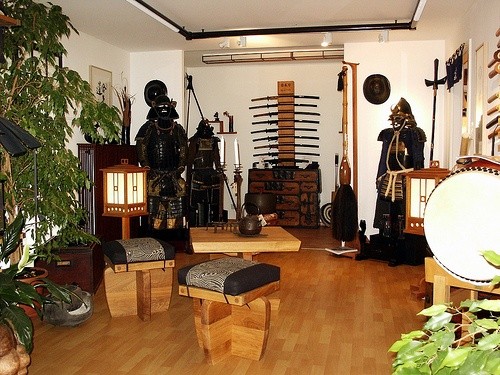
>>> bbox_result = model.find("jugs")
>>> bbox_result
[238,215,263,235]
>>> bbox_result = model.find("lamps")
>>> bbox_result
[403,160,451,236]
[219,29,390,49]
[99,158,150,238]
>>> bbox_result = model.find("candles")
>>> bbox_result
[233,138,240,164]
[221,136,225,162]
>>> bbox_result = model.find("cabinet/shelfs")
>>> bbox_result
[248,80,320,227]
[424,256,500,347]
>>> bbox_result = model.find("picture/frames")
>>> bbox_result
[88,64,113,109]
[11,38,63,108]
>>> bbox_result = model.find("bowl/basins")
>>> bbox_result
[245,193,275,215]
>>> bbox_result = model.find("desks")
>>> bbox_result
[190,227,302,261]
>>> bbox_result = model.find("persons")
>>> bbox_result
[135,95,189,251]
[373,98,427,267]
[187,119,222,227]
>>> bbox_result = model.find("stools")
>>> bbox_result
[178,256,281,365]
[103,235,176,322]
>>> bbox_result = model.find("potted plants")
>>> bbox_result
[0,0,102,375]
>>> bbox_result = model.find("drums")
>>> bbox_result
[423,159,500,285]
